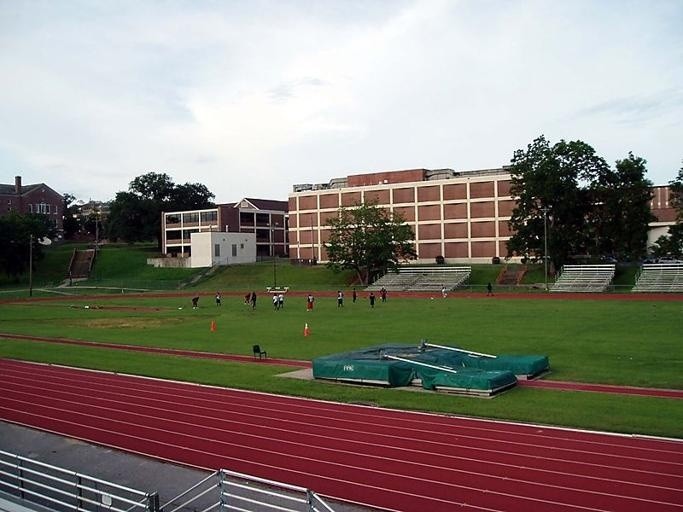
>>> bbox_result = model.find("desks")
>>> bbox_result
[289,257,317,266]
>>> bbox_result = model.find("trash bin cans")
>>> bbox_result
[493,257,499,264]
[436,256,444,264]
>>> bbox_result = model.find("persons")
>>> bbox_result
[440,283,447,298]
[484,281,494,297]
[249,290,256,311]
[271,293,279,313]
[305,294,314,312]
[379,286,387,298]
[213,292,221,307]
[244,293,250,305]
[367,292,376,311]
[278,293,284,309]
[380,291,386,303]
[351,288,357,304]
[191,296,199,307]
[336,290,344,309]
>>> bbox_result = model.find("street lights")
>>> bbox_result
[538,205,552,293]
[266,223,278,287]
